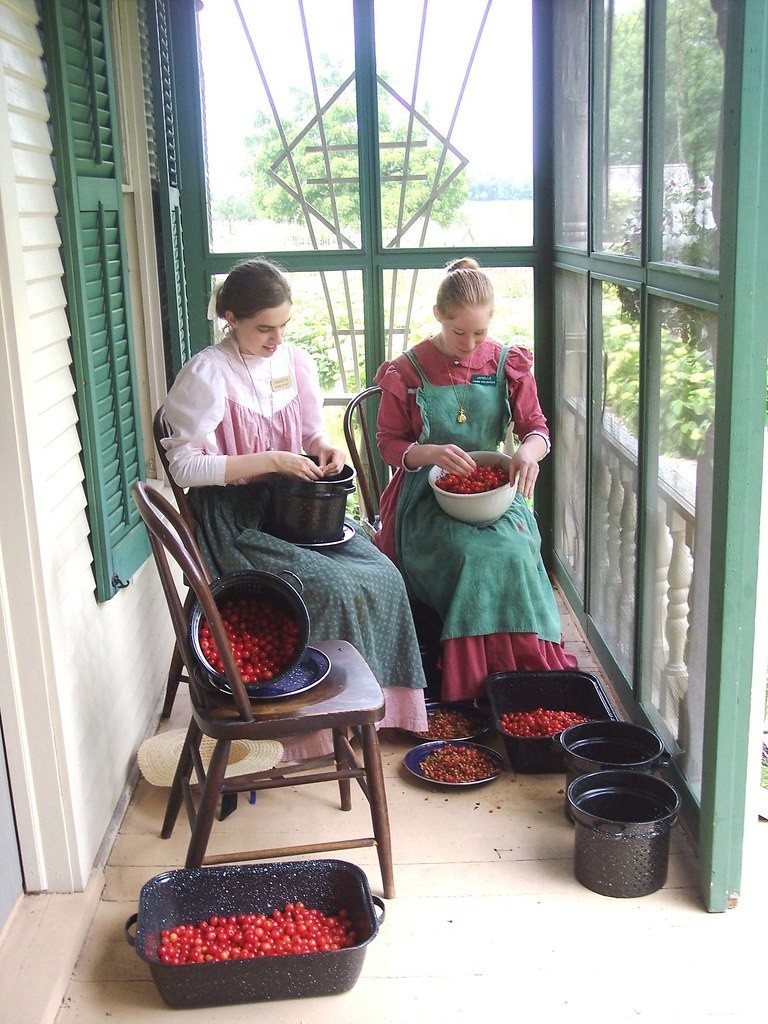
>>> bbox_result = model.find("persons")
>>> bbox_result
[160,259,429,762]
[376,256,578,703]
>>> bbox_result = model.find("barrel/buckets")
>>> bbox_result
[270,456,358,542]
[566,768,682,898]
[190,568,310,694]
[552,719,672,824]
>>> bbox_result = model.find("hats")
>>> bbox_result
[137,727,284,788]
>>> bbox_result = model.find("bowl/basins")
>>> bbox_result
[428,451,521,528]
[125,859,385,1009]
[484,670,620,772]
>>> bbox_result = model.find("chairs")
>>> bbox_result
[152,403,200,713]
[342,384,396,524]
[132,480,399,902]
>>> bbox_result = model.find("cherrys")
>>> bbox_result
[499,705,598,737]
[435,459,510,494]
[200,597,300,683]
[158,900,357,964]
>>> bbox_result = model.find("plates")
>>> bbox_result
[261,519,357,547]
[412,701,492,739]
[405,740,505,786]
[193,645,333,698]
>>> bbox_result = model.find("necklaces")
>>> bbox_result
[444,356,473,423]
[245,356,277,451]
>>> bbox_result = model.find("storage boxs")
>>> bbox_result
[123,858,385,1009]
[487,667,622,775]
